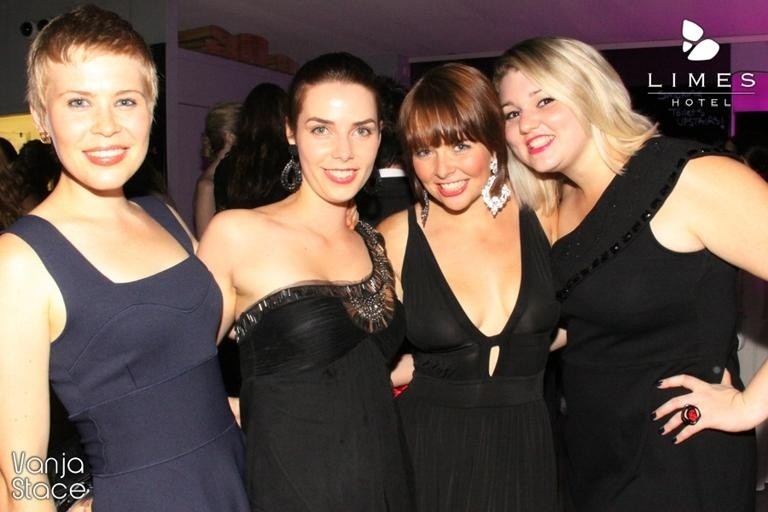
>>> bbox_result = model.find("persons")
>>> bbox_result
[360,60,573,512]
[487,34,768,510]
[191,100,239,242]
[186,48,425,512]
[0,0,266,511]
[0,133,61,239]
[664,115,768,183]
[351,75,434,234]
[210,79,303,432]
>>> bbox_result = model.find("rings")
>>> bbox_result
[681,405,701,425]
